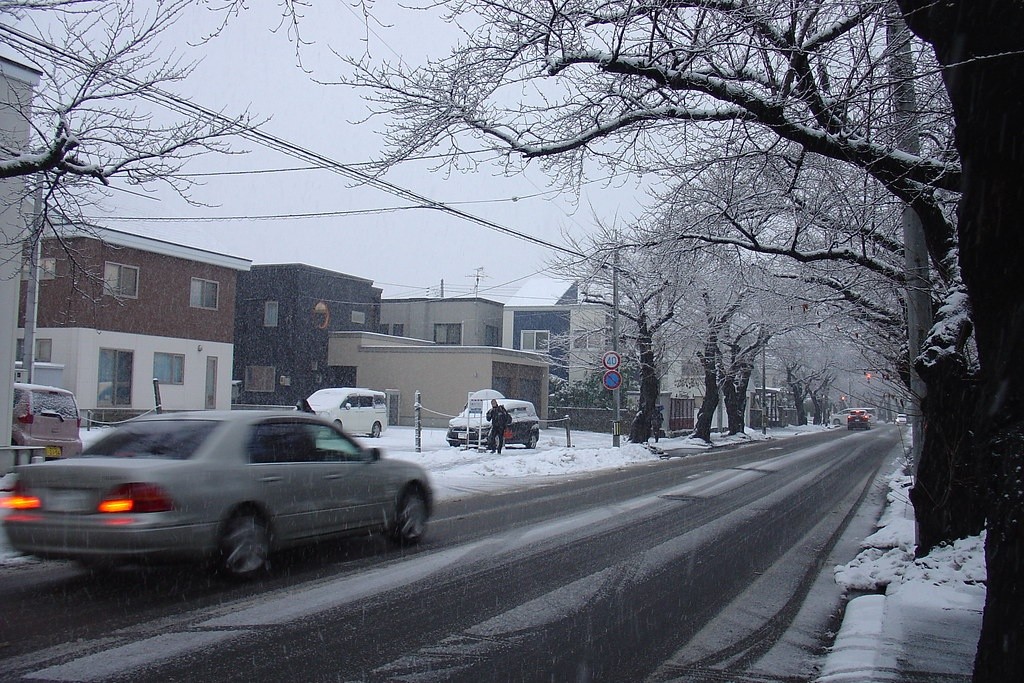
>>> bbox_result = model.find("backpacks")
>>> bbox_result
[492,405,512,424]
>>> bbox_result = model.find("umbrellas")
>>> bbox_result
[654,404,665,411]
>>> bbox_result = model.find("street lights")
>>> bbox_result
[842,377,852,407]
[611,240,642,450]
[762,334,774,435]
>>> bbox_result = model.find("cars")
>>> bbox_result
[296,387,388,438]
[830,407,877,427]
[4,410,435,581]
[892,417,896,422]
[897,414,907,425]
[12,382,84,462]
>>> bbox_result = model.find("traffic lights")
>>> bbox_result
[866,373,871,384]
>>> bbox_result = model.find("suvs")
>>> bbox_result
[848,409,872,430]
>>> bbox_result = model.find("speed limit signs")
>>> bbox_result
[602,351,620,369]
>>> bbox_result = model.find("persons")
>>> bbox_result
[295,399,319,440]
[486,399,509,454]
[651,407,664,443]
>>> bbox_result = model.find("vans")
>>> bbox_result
[446,389,539,449]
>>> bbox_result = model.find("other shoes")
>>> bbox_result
[490,450,496,453]
[498,450,501,454]
